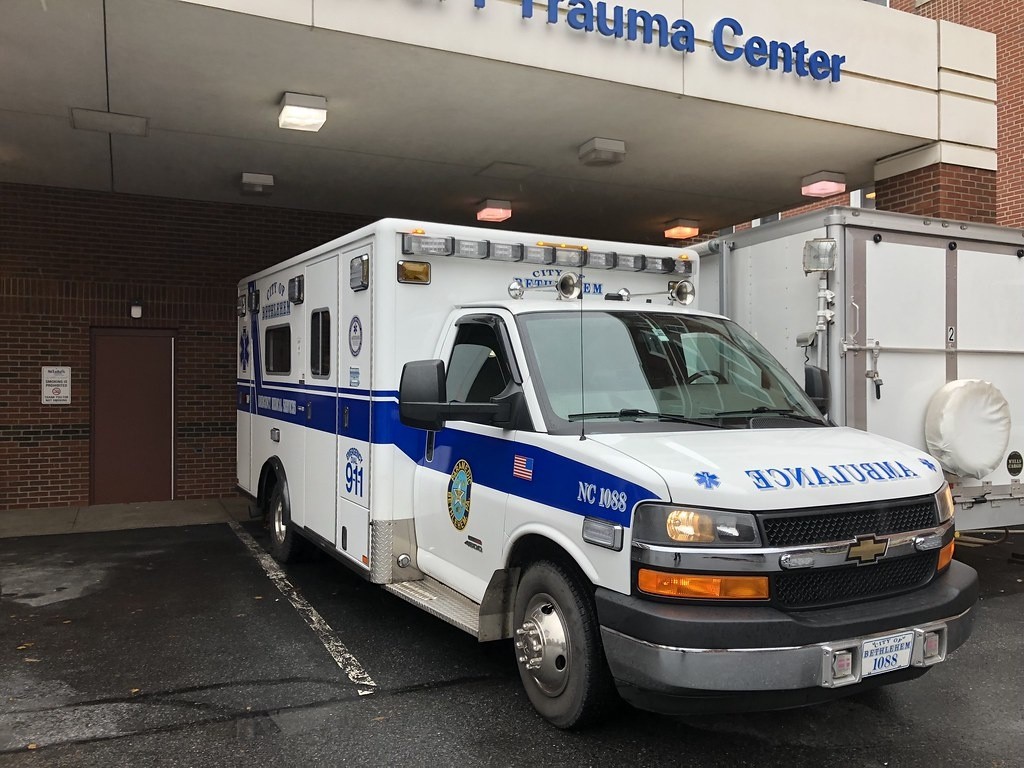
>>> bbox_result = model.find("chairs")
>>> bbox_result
[601,322,671,403]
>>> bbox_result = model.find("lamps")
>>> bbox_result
[664,219,698,239]
[240,173,274,197]
[801,171,846,198]
[476,200,511,223]
[277,92,329,133]
[578,136,627,166]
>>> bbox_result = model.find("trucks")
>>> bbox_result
[683,204,1024,543]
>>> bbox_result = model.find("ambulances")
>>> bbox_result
[239,218,980,730]
